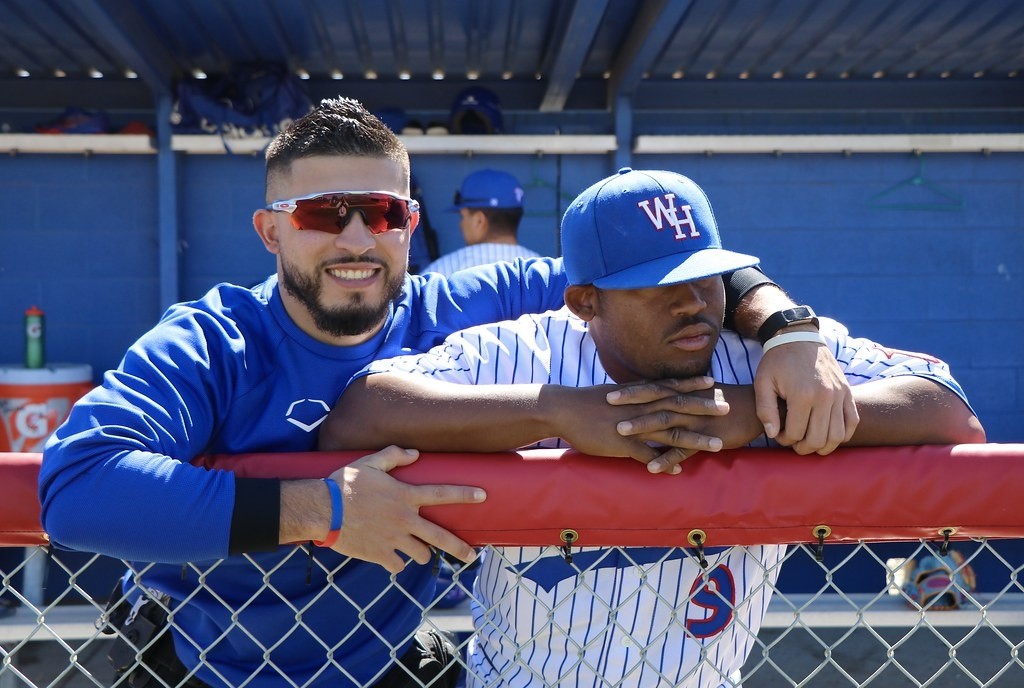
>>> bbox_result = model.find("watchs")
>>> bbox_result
[757,302,820,344]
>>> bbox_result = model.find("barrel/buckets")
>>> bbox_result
[0,363,93,454]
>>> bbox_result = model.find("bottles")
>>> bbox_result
[25,306,45,369]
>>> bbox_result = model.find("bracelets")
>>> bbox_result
[312,478,344,546]
[763,330,829,357]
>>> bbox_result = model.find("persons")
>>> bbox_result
[319,163,986,688]
[36,98,859,688]
[420,168,546,278]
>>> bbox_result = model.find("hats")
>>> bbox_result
[560,167,760,291]
[443,171,525,211]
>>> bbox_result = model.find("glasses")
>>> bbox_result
[454,190,492,206]
[264,190,421,234]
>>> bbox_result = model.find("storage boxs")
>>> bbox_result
[0,363,93,453]
